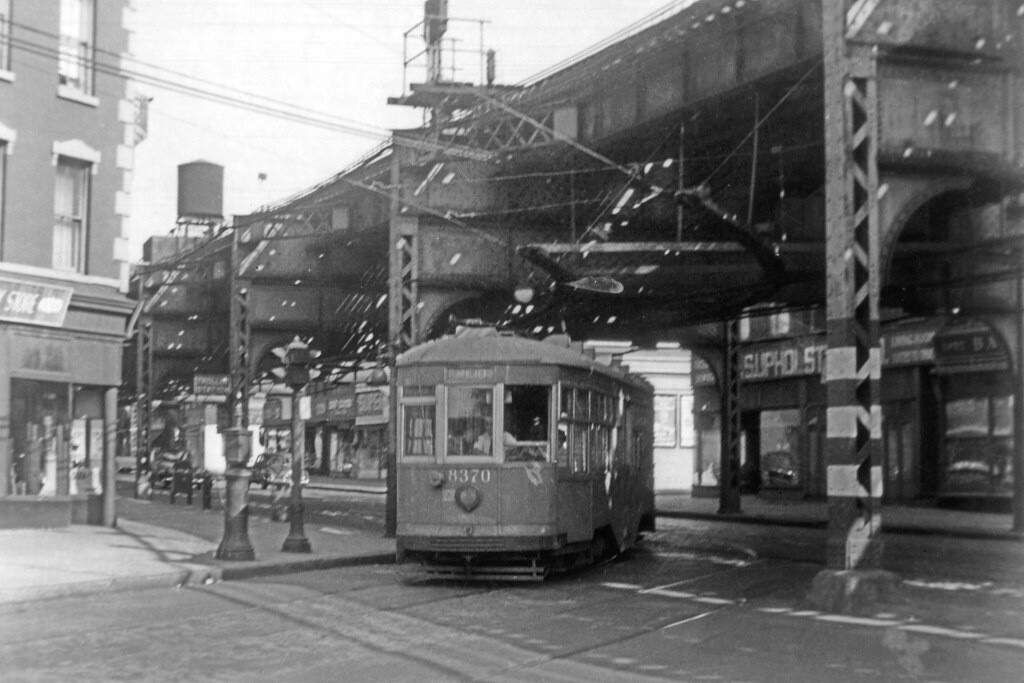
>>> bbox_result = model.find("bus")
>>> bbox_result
[393,327,655,580]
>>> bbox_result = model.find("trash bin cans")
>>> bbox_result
[168,460,194,506]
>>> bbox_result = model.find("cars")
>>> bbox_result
[252,453,310,490]
[149,448,215,491]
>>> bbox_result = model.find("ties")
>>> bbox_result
[489,439,493,455]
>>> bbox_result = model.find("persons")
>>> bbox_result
[474,414,516,456]
